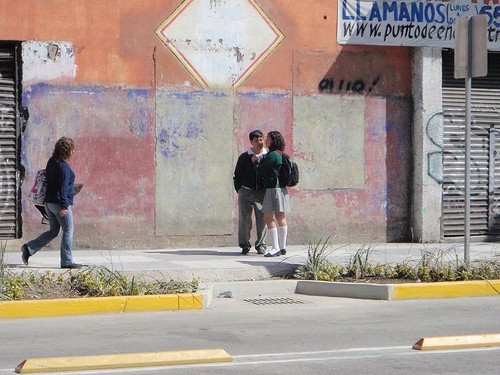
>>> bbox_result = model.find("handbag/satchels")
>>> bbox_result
[33,170,50,222]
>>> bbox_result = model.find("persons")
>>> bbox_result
[252,131,289,257]
[21,136,83,269]
[233,130,270,255]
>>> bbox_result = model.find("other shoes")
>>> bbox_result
[242,248,250,254]
[61,263,83,269]
[257,247,265,254]
[281,248,286,255]
[21,243,29,265]
[264,250,281,257]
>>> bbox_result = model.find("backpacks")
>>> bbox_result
[277,149,298,187]
